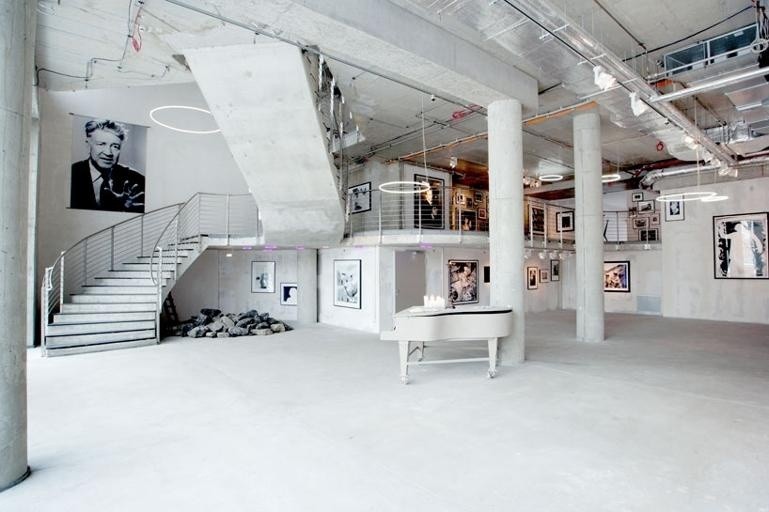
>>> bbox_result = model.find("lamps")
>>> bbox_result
[591,66,739,178]
[449,157,457,168]
[523,176,542,189]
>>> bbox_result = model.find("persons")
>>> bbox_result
[415,187,442,228]
[460,215,473,230]
[718,220,764,278]
[70,116,146,213]
[605,264,626,289]
[529,270,537,286]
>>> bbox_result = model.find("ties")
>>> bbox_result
[99,174,110,202]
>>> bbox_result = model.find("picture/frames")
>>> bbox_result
[447,189,575,306]
[604,260,631,293]
[665,193,685,222]
[280,284,298,306]
[629,192,660,241]
[346,181,372,214]
[250,261,276,294]
[413,174,446,229]
[713,212,769,280]
[333,258,362,309]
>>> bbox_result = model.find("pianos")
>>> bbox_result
[392,304,513,385]
